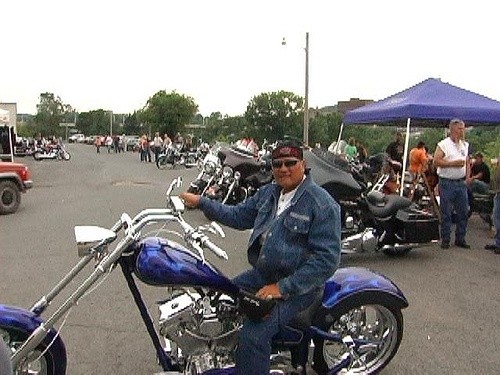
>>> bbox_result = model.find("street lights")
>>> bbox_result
[281,32,310,146]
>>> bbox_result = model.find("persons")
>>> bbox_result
[434,119,471,248]
[346,119,500,215]
[484,153,500,254]
[35,130,196,163]
[179,146,341,375]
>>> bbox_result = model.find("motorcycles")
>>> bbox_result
[186,139,275,206]
[32,144,71,160]
[0,176,409,375]
[157,142,199,170]
[301,146,500,258]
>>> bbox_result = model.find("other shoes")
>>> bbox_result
[455,241,470,248]
[485,244,500,254]
[441,242,449,249]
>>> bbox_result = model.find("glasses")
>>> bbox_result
[272,159,301,168]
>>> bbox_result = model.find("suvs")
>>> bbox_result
[68,134,140,150]
[0,158,33,213]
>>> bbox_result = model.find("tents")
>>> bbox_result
[334,77,500,197]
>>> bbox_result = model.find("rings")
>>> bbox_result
[267,295,272,300]
